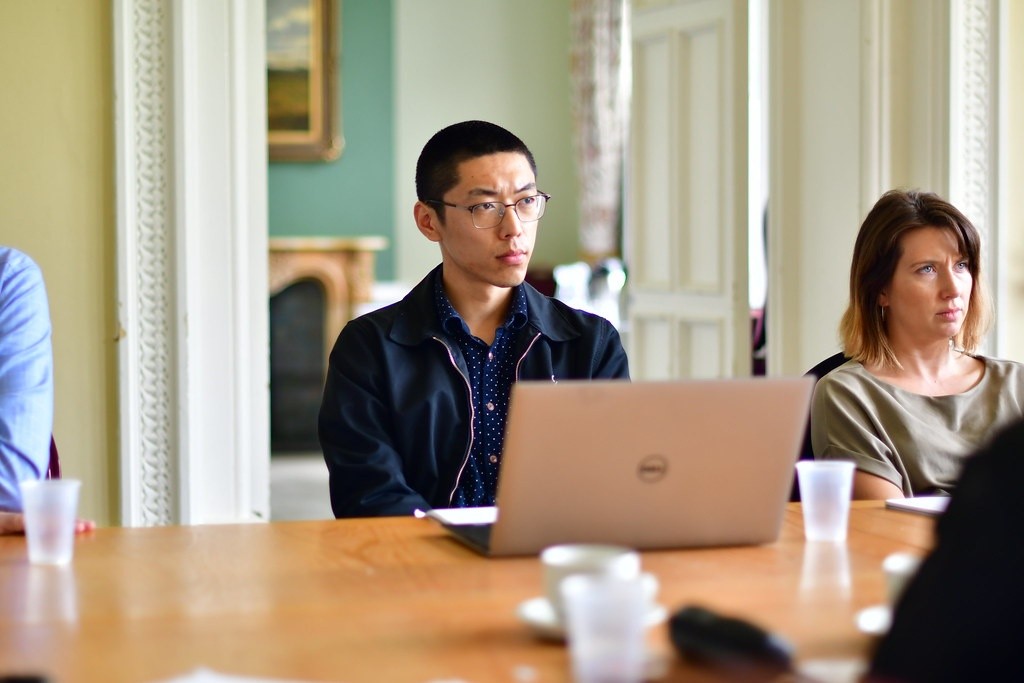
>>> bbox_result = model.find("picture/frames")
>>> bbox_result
[266,0,345,161]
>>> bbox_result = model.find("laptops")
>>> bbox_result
[429,372,816,557]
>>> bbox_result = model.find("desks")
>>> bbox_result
[0,503,934,682]
[269,236,386,380]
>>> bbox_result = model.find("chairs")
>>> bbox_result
[792,351,852,500]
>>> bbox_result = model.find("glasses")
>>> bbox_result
[425,189,550,229]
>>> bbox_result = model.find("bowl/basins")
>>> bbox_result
[539,541,641,626]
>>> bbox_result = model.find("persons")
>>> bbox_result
[319,120,631,521]
[638,409,1024,683]
[811,186,1024,500]
[0,245,96,533]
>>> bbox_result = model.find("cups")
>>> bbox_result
[559,569,658,682]
[19,478,84,567]
[794,460,856,546]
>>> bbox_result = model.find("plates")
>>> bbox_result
[515,597,671,642]
